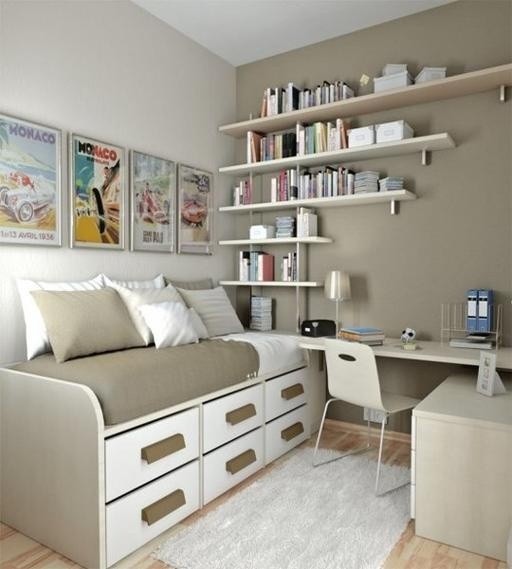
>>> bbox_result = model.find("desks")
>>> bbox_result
[410,374,512,563]
[299,336,512,371]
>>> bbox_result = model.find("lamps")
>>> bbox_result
[324,270,352,339]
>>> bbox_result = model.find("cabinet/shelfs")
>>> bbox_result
[218,63,512,333]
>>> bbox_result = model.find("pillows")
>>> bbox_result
[15,274,245,362]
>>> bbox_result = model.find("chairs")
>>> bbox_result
[311,339,422,497]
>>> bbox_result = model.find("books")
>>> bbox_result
[238,250,298,282]
[449,288,494,350]
[248,297,274,331]
[338,326,383,350]
[227,61,445,243]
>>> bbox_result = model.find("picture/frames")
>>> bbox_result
[0,113,62,247]
[176,162,215,255]
[129,149,176,253]
[68,132,125,250]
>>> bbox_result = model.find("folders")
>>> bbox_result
[467,289,493,333]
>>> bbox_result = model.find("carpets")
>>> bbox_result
[152,445,411,569]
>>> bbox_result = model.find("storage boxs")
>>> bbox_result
[415,67,447,84]
[346,125,374,147]
[373,71,414,92]
[374,119,414,143]
[382,64,407,77]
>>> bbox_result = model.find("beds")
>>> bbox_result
[0,332,327,569]
